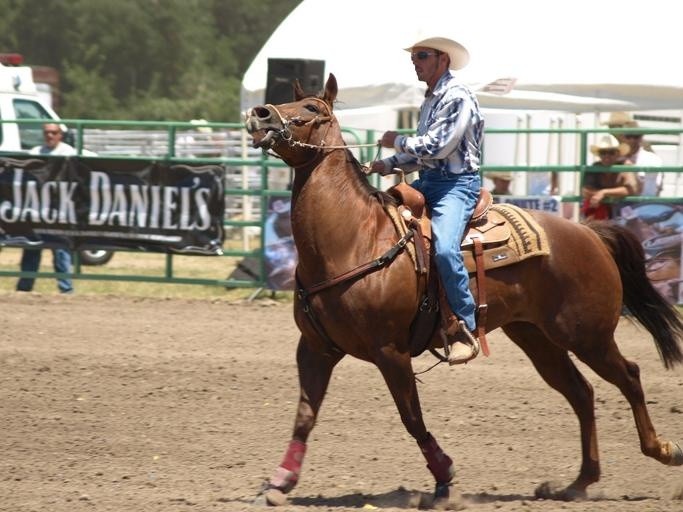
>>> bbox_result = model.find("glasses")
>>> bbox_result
[411,49,439,61]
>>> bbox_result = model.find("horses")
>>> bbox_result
[242,70,683,512]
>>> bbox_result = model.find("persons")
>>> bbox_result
[362,36,485,364]
[18,123,77,292]
[580,112,662,209]
[486,170,515,195]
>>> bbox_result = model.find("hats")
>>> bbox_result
[402,37,470,70]
[590,112,648,157]
[483,172,520,181]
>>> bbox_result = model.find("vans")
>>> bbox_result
[0,53,114,265]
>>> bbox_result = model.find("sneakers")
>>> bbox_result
[448,329,479,362]
